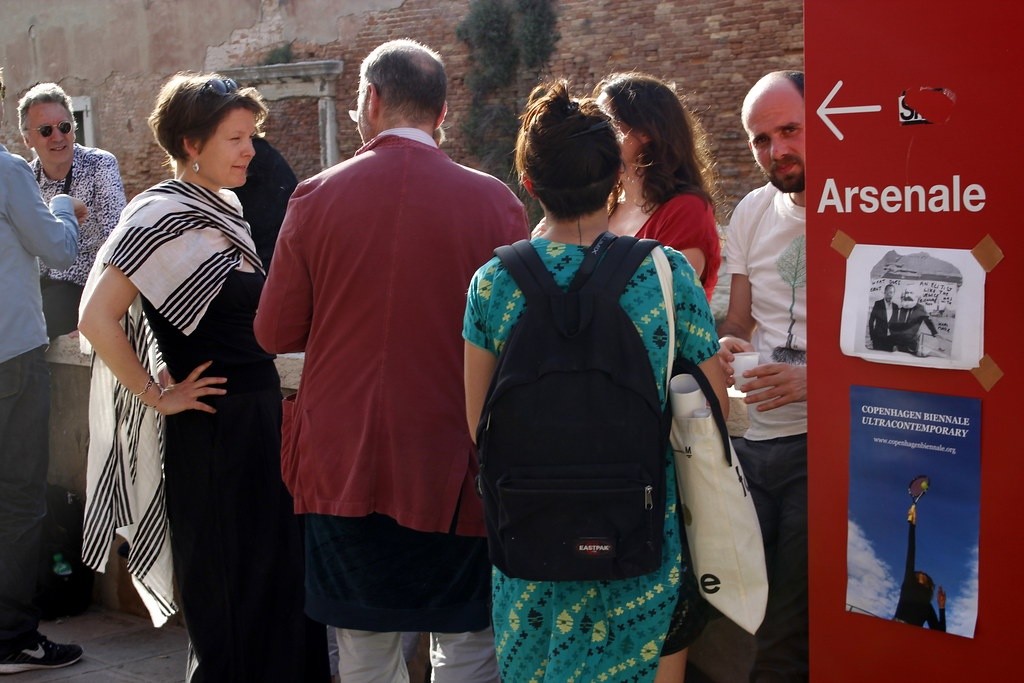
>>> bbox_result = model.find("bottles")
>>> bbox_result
[52,553,73,575]
[694,410,709,418]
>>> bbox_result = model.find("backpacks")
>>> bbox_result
[474,231,669,582]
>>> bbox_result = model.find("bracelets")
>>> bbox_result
[144,383,163,407]
[135,376,154,396]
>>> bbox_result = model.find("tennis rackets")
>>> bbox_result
[908,472,930,525]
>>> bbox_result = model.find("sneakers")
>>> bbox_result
[0,632,84,673]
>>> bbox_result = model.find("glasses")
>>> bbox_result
[23,121,72,138]
[347,90,369,122]
[186,78,238,127]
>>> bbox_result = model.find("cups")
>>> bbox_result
[730,351,760,389]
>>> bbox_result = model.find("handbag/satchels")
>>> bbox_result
[667,360,769,635]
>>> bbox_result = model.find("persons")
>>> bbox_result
[16,83,127,342]
[77,71,334,683]
[0,66,86,672]
[531,73,722,683]
[895,510,947,632]
[869,285,937,356]
[253,40,530,683]
[719,70,809,683]
[462,77,729,683]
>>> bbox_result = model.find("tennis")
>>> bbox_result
[920,481,929,490]
